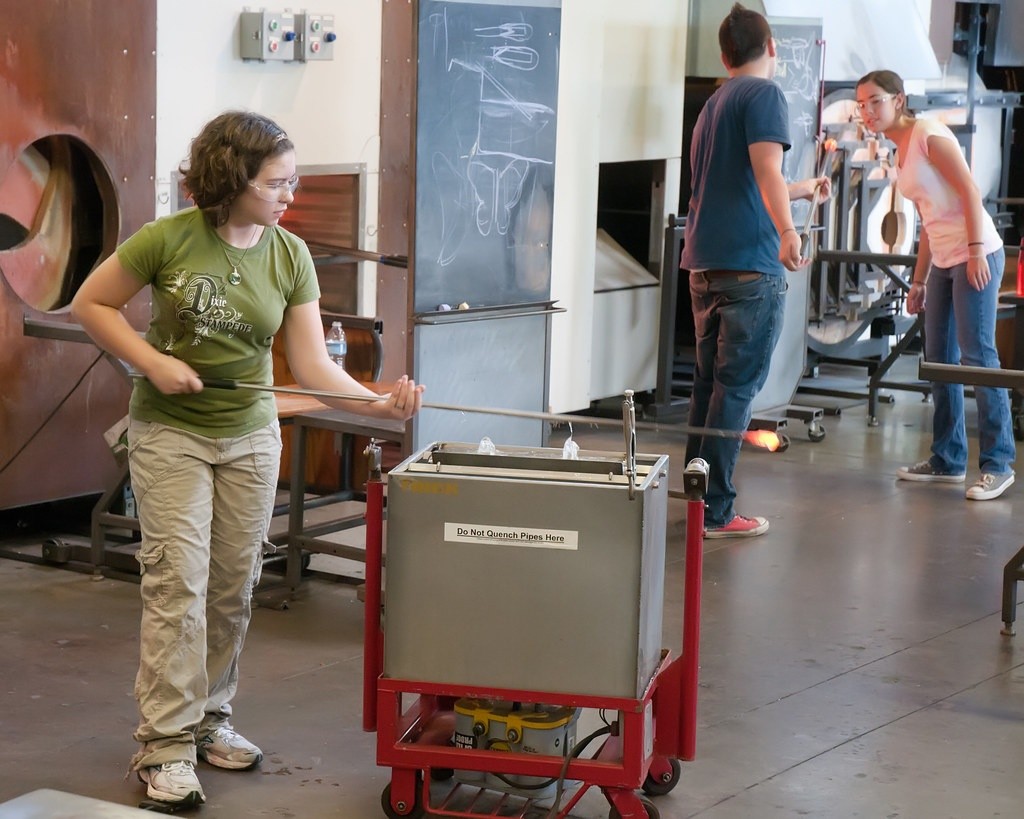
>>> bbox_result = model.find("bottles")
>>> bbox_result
[326,321,348,376]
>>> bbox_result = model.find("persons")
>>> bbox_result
[67,111,426,809]
[855,68,1015,501]
[679,0,834,537]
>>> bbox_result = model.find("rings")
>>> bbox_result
[395,404,404,410]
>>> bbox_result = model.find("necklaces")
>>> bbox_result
[213,224,258,286]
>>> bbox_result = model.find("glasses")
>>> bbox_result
[242,176,299,200]
[854,94,895,112]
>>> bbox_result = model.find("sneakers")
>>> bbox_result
[896,458,966,483]
[698,509,768,540]
[966,468,1015,500]
[196,728,264,771]
[136,758,207,810]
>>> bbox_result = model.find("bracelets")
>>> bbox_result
[967,241,985,246]
[912,280,926,286]
[780,226,795,238]
[968,253,986,258]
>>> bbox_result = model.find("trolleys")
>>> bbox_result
[362,439,698,818]
